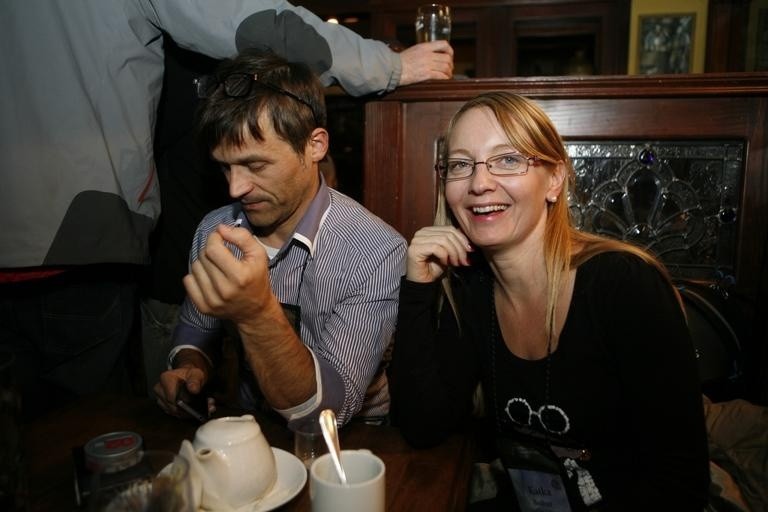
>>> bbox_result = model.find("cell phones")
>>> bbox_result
[175,382,209,424]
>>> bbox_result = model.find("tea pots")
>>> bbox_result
[181,412,279,508]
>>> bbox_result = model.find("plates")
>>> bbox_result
[153,443,309,511]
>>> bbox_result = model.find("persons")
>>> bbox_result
[638,13,694,75]
[0,0,457,430]
[379,87,714,511]
[150,46,411,447]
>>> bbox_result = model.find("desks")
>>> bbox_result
[27,393,476,512]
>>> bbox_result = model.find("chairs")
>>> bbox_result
[671,274,758,404]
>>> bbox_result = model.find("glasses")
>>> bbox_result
[435,153,545,179]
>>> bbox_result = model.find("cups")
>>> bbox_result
[416,4,455,53]
[308,443,391,512]
